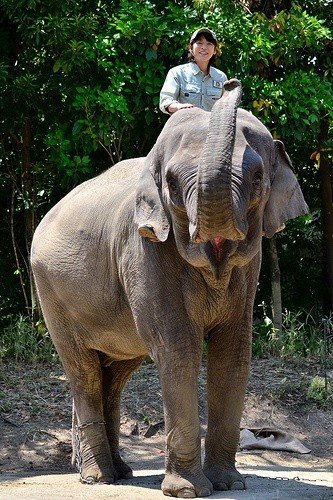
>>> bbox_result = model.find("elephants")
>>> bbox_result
[28,79,310,499]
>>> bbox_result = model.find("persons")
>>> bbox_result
[160,27,228,116]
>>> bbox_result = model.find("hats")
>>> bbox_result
[190,27,217,44]
